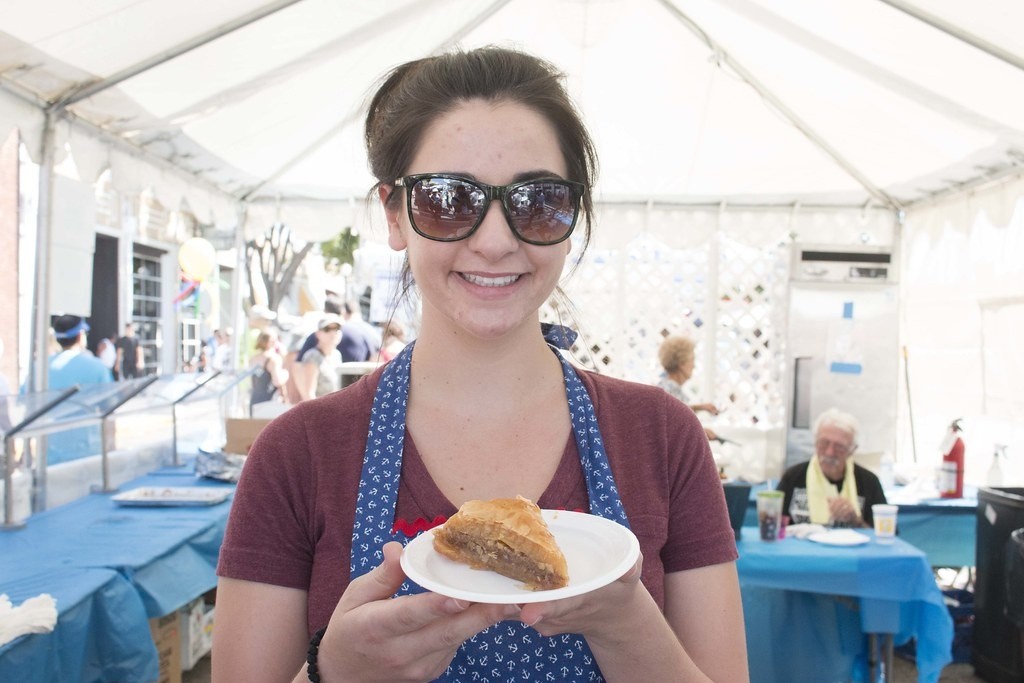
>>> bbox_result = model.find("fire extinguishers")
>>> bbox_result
[939,418,966,499]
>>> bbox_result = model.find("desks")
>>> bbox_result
[0,449,237,683]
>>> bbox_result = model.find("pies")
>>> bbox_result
[431,497,569,591]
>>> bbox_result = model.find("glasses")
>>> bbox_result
[324,324,342,333]
[383,173,584,246]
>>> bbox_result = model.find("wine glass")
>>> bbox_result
[790,488,811,539]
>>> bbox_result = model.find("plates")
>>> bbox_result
[109,488,234,506]
[805,529,871,545]
[400,509,639,605]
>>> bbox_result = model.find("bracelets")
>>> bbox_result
[306,625,328,683]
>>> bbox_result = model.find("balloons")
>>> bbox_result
[178,237,217,280]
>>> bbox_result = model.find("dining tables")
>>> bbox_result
[734,526,927,683]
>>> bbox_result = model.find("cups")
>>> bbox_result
[847,495,865,527]
[872,503,899,545]
[757,490,789,538]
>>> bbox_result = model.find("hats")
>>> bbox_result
[252,303,278,321]
[318,313,345,330]
[52,316,89,339]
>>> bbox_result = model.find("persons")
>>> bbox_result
[115,323,145,379]
[97,332,117,369]
[205,47,750,683]
[413,182,478,219]
[656,334,718,441]
[774,410,899,536]
[187,326,233,373]
[512,189,522,215]
[535,189,545,207]
[250,293,407,420]
[0,315,116,472]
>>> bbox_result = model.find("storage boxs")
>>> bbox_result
[222,416,275,455]
[149,596,215,683]
